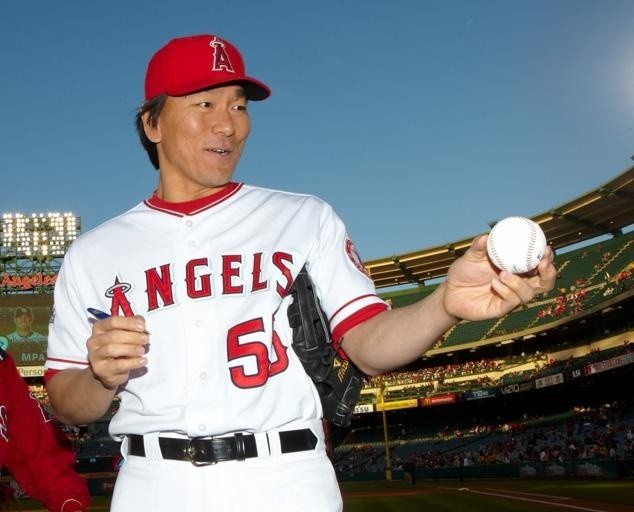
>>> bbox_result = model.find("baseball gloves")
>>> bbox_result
[289,266,366,431]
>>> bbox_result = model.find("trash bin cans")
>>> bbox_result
[405,472,413,484]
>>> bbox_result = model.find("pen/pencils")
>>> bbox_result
[87,307,153,337]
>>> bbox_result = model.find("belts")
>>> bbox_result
[126,428,319,467]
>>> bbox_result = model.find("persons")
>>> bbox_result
[46,414,123,473]
[0,347,91,511]
[1,306,49,365]
[44,34,558,512]
[526,249,633,322]
[355,335,633,405]
[332,367,632,485]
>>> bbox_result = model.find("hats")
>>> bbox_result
[143,34,272,103]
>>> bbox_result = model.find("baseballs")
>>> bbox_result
[486,216,547,276]
